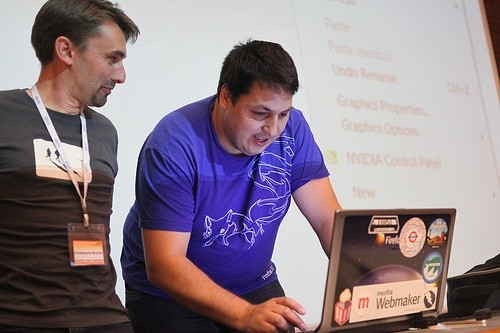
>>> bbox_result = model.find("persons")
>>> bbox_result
[0,0,140,333]
[120,39,344,333]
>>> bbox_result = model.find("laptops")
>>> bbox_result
[299,207,456,333]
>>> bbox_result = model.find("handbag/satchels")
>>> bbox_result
[410,253,500,329]
